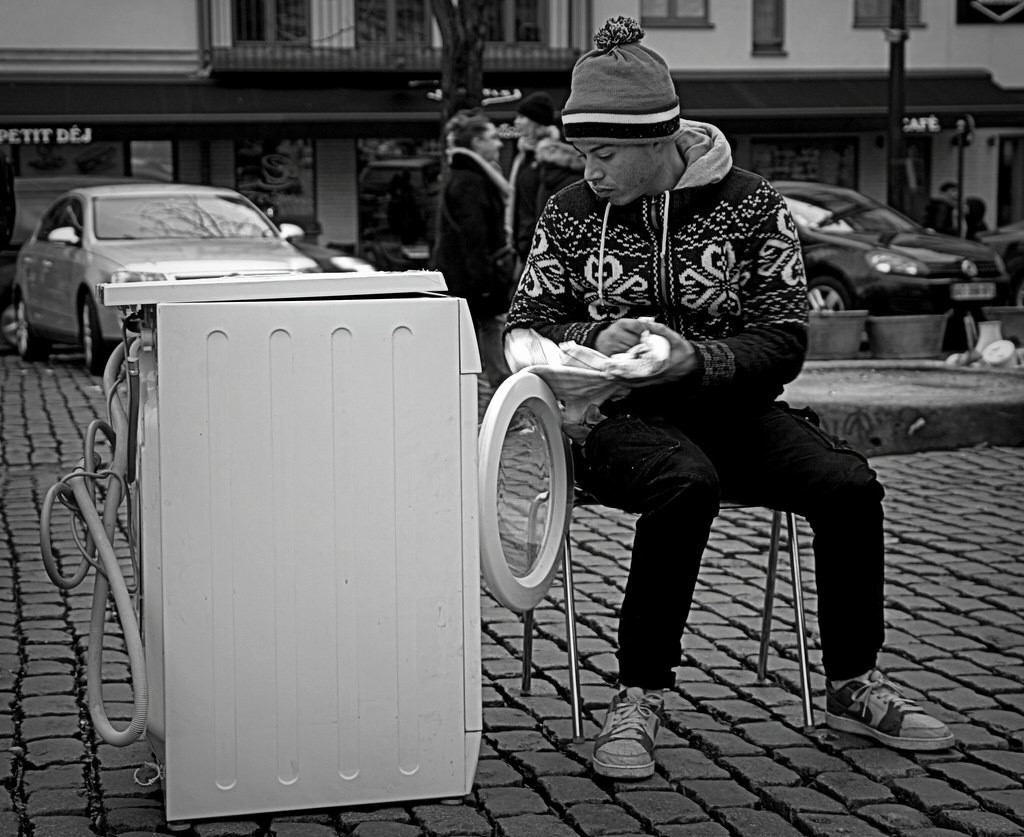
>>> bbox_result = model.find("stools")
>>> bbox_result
[520,485,815,745]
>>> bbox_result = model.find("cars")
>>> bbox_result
[12,182,323,376]
[972,218,1024,311]
[288,238,377,273]
[766,178,1012,316]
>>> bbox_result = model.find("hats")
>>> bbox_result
[558,15,681,143]
[517,91,556,126]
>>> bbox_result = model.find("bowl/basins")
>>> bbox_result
[981,306,1024,348]
[804,309,869,360]
[869,308,954,359]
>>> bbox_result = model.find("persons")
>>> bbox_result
[503,18,956,778]
[921,181,988,242]
[434,92,588,432]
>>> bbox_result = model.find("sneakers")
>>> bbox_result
[822,669,955,749]
[592,687,664,778]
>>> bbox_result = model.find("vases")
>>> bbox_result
[983,305,1024,348]
[806,309,868,360]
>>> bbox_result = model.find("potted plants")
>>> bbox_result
[861,281,956,360]
